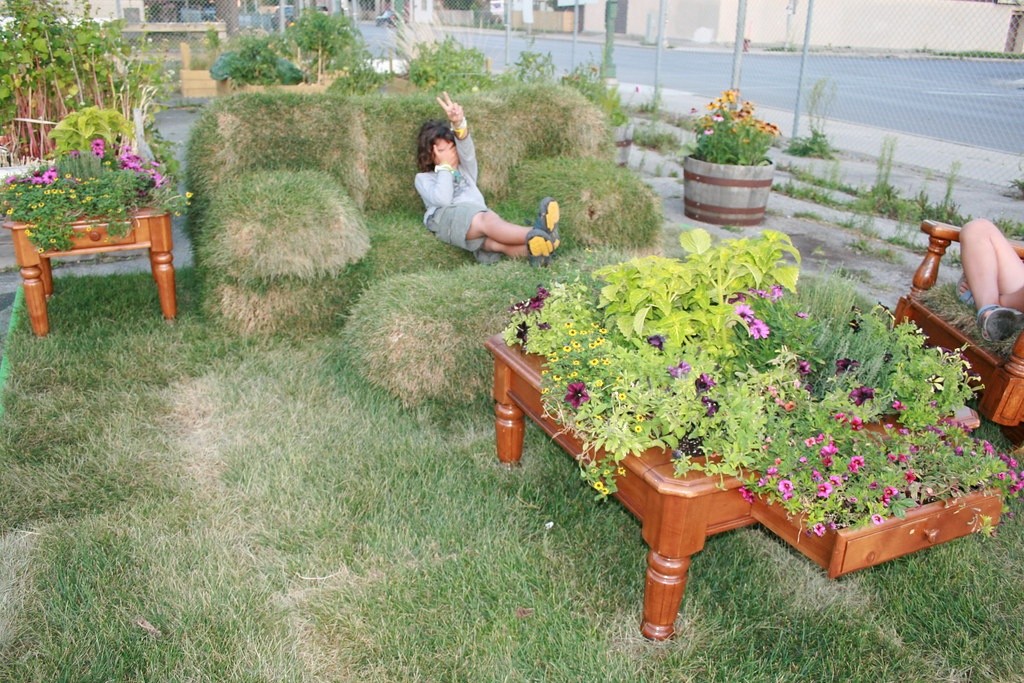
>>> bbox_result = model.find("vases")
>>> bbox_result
[611,123,634,166]
[684,153,775,226]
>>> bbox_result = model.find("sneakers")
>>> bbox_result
[526,228,552,268]
[532,196,561,251]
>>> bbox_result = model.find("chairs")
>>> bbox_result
[891,220,1023,444]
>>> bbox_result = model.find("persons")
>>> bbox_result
[955,218,1024,342]
[414,91,560,267]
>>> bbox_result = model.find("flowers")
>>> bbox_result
[688,85,782,164]
[0,133,194,253]
[598,81,640,126]
[502,227,1024,543]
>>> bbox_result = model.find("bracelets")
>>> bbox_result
[434,163,455,172]
[450,118,467,138]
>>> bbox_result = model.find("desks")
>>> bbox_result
[482,328,1005,639]
[0,206,176,336]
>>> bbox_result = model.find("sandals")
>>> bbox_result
[956,276,976,309]
[974,304,1024,343]
